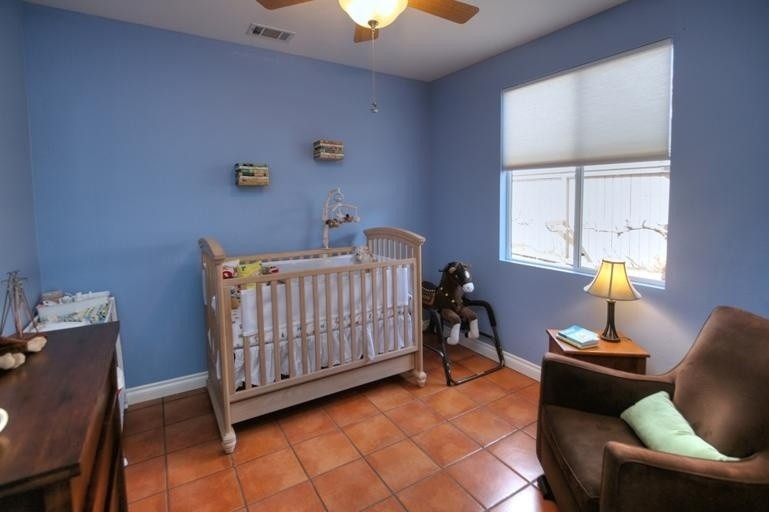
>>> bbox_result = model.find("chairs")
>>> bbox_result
[535,304,769,512]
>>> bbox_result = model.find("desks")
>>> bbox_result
[0,322,128,512]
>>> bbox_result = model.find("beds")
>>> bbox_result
[197,222,427,453]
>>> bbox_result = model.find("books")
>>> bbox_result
[558,324,600,345]
[556,334,600,350]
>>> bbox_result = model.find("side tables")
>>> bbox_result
[545,329,650,375]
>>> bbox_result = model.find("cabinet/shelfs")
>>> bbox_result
[23,297,128,435]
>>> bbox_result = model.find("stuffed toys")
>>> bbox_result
[0,333,48,371]
[354,245,378,272]
[222,261,286,310]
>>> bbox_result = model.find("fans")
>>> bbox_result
[255,0,480,43]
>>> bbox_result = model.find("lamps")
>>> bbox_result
[582,258,642,342]
[338,0,408,114]
